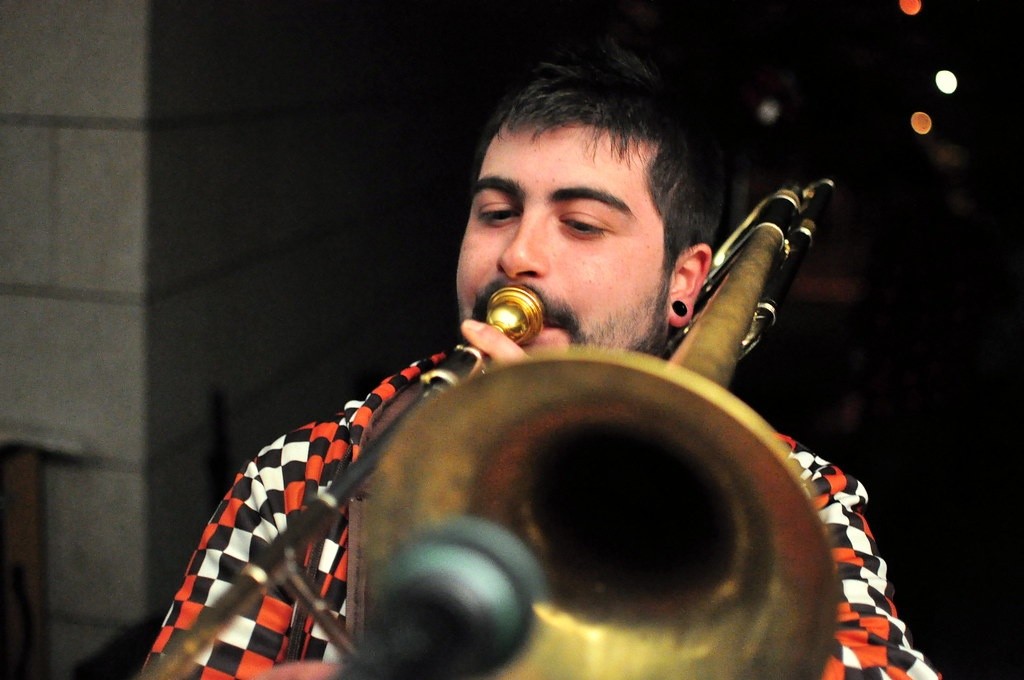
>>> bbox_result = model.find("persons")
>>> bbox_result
[143,40,943,680]
[726,60,881,438]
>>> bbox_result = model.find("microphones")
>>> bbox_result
[360,518,550,680]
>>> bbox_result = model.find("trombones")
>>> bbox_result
[126,170,846,678]
